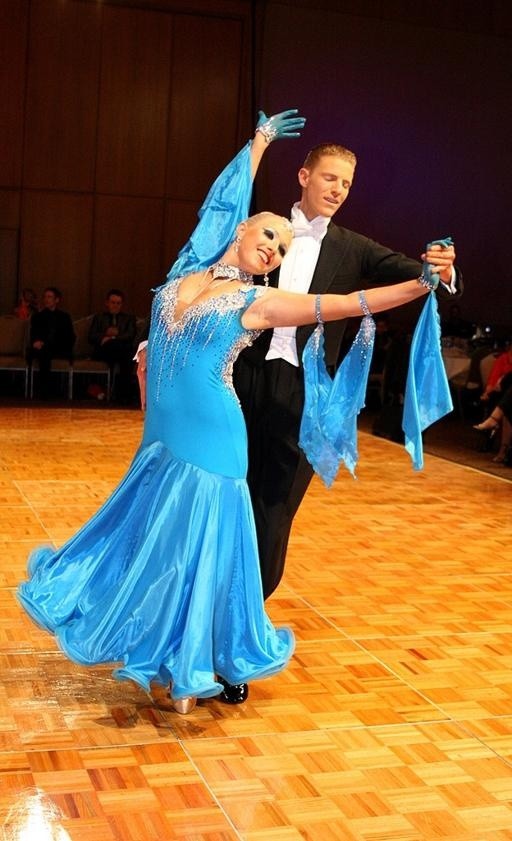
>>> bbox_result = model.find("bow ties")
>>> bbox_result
[287,216,330,244]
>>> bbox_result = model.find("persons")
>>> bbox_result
[14,108,458,716]
[86,287,136,408]
[344,315,509,475]
[29,287,77,405]
[14,288,38,319]
[131,135,456,716]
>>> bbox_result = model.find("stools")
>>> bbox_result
[29,353,72,401]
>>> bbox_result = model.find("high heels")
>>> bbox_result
[471,415,501,440]
[490,442,511,465]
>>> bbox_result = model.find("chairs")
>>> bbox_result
[0,314,30,401]
[474,348,501,420]
[366,355,386,405]
[71,314,111,404]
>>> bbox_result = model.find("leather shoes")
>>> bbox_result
[215,671,250,706]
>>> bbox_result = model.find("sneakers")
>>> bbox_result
[170,694,200,717]
